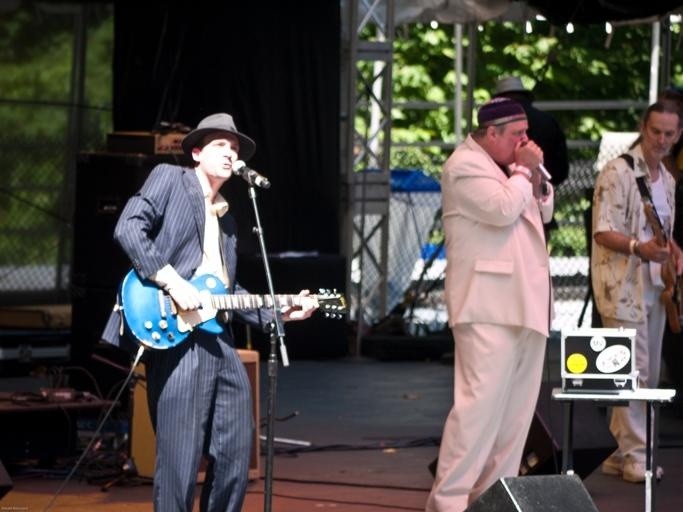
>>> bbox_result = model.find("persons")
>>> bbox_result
[591,100,682,482]
[492,77,570,250]
[629,90,682,246]
[424,98,555,511]
[98,113,322,510]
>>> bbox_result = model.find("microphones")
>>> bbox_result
[232,160,272,190]
[521,141,552,181]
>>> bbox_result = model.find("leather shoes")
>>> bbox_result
[602,456,662,482]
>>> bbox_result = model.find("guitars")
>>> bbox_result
[119,264,347,353]
[641,198,683,334]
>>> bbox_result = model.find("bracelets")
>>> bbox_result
[628,237,640,255]
[512,165,532,178]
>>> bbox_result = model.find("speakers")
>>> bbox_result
[68,150,189,363]
[463,474,599,512]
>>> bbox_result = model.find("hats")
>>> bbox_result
[477,99,527,129]
[181,113,256,162]
[490,76,535,103]
